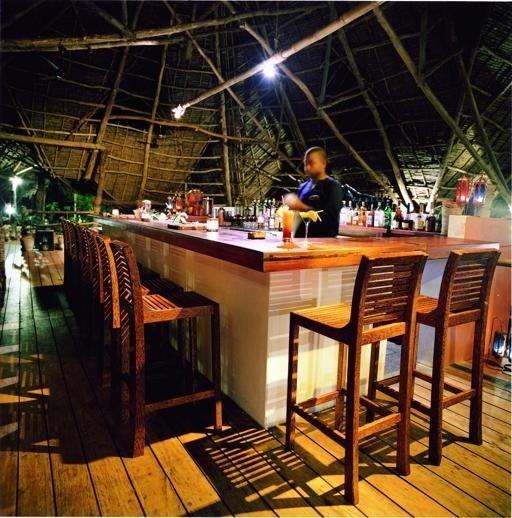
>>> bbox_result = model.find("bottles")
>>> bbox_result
[340,199,442,232]
[249,198,277,221]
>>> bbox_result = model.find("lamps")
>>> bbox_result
[485,317,506,370]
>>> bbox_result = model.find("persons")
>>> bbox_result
[282,147,341,238]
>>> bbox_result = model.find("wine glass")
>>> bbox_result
[175,197,183,214]
[298,210,313,245]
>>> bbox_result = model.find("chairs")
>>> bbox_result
[60,217,90,296]
[366,249,501,465]
[96,233,187,389]
[285,251,428,503]
[85,226,160,325]
[109,240,223,457]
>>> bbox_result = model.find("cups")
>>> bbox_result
[207,218,219,232]
[282,210,294,243]
